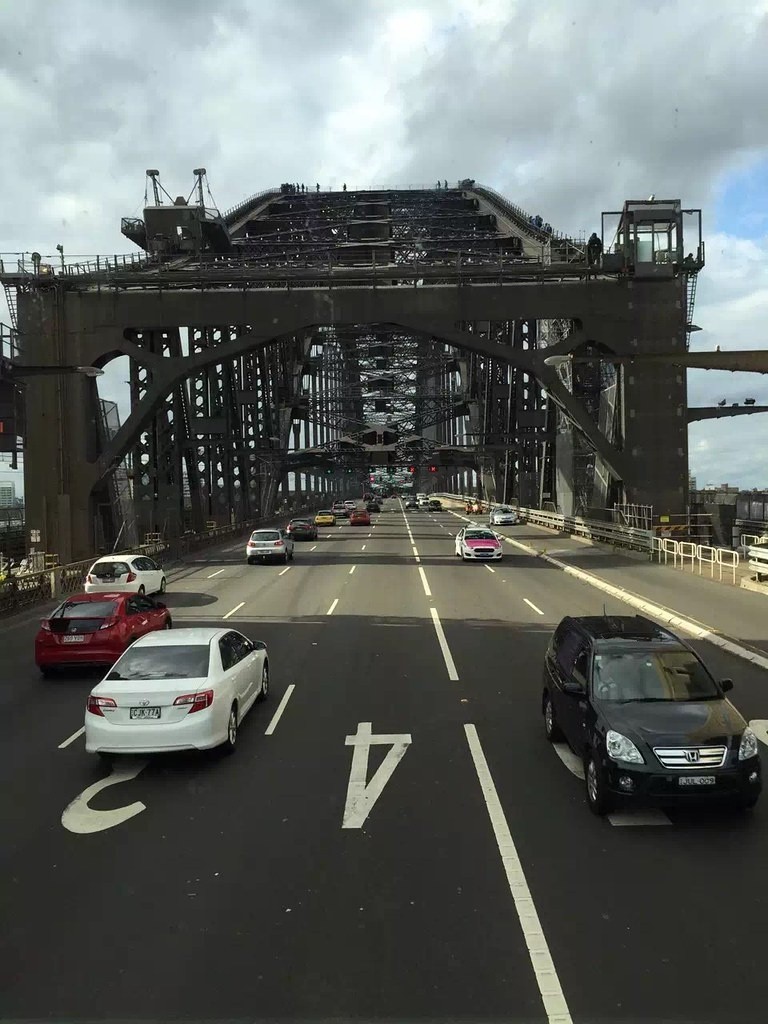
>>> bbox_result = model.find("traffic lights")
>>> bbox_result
[346,467,353,473]
[407,466,419,472]
[428,466,438,472]
[386,467,396,473]
[324,468,332,474]
[367,466,375,472]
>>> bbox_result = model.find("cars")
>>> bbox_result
[540,615,764,819]
[489,507,516,525]
[34,592,174,678]
[454,525,502,560]
[83,628,269,758]
[245,528,297,562]
[286,517,319,541]
[85,554,167,596]
[315,492,444,528]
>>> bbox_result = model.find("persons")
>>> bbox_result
[588,233,603,266]
[437,178,475,190]
[280,182,347,193]
[529,214,552,233]
[683,253,695,283]
